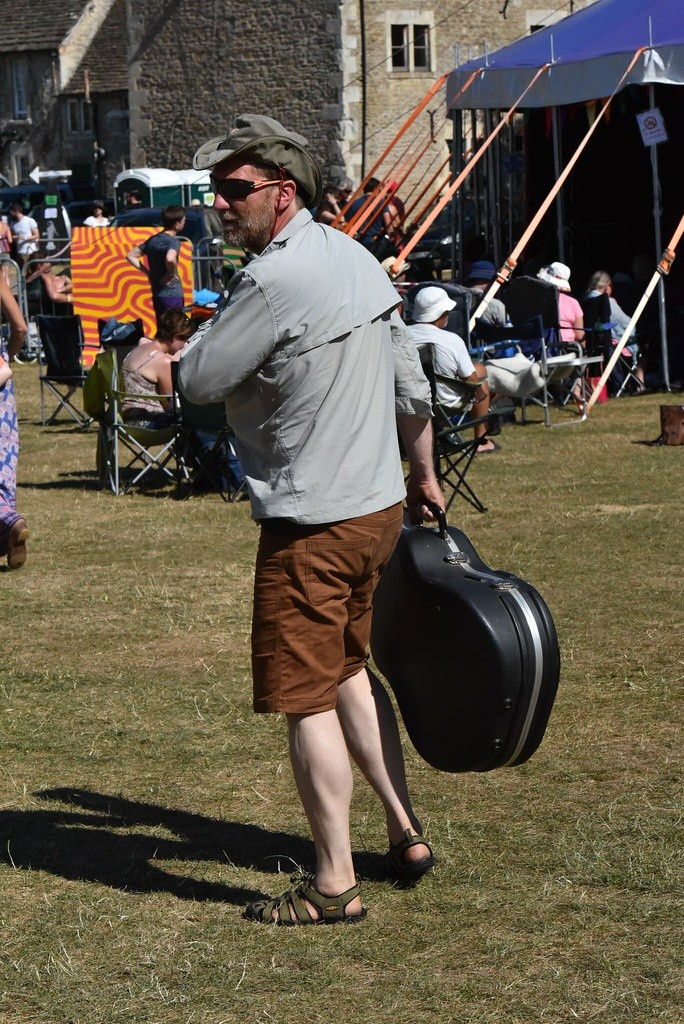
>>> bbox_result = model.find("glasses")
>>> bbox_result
[178,313,189,335]
[208,174,287,200]
[343,188,352,195]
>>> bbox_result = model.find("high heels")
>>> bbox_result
[7,517,30,570]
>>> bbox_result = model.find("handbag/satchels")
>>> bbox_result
[582,377,607,405]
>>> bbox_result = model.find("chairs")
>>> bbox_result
[34,274,647,514]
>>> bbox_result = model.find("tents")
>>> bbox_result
[447,0,684,388]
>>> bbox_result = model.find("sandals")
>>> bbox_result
[245,884,368,925]
[380,827,437,876]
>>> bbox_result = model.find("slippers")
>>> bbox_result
[477,439,502,453]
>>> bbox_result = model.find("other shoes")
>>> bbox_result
[573,392,587,416]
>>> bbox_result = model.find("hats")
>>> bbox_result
[537,262,571,292]
[193,113,322,210]
[90,200,107,212]
[463,261,496,280]
[381,256,411,280]
[412,284,458,320]
[385,179,398,193]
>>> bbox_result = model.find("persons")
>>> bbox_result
[465,262,513,333]
[406,287,502,453]
[125,188,146,209]
[83,201,111,227]
[0,204,39,265]
[171,114,444,927]
[537,261,586,397]
[28,251,72,302]
[119,308,244,489]
[586,270,644,392]
[126,205,186,320]
[316,177,405,261]
[0,276,29,568]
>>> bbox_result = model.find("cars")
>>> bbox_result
[0,166,226,292]
[402,189,521,285]
